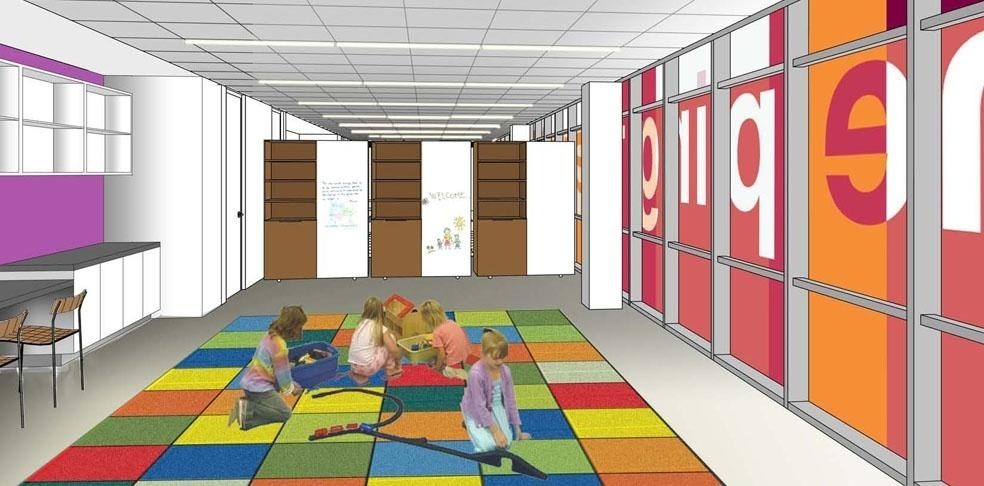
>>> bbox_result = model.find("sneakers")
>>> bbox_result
[442,365,468,381]
[387,367,404,377]
[236,398,248,431]
[228,401,238,428]
[350,369,370,385]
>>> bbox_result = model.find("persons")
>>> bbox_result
[419,299,472,378]
[347,297,399,385]
[459,329,530,453]
[227,306,308,430]
